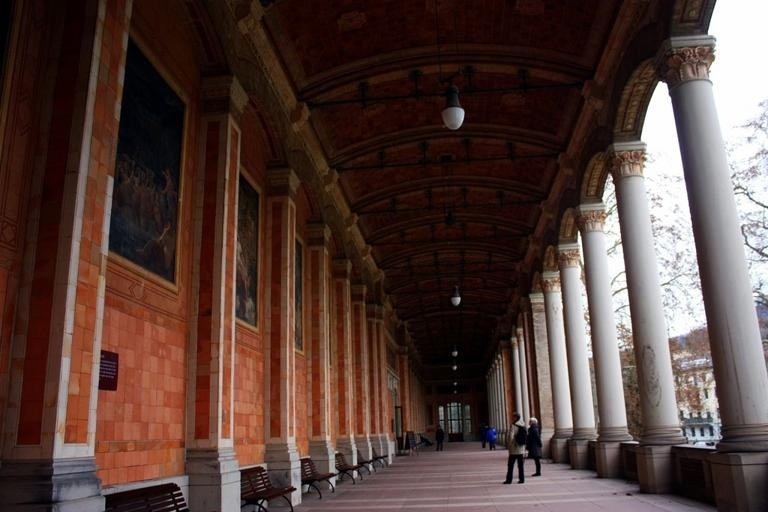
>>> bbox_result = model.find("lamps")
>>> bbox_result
[433,0,470,132]
[450,283,462,308]
[450,339,458,358]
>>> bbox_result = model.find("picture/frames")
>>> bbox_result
[295,233,305,358]
[234,165,262,336]
[107,28,190,298]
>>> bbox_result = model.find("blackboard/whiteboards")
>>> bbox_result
[407,431,416,448]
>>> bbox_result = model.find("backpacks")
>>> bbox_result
[512,422,527,446]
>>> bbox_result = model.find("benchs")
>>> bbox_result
[103,482,191,512]
[357,448,372,479]
[335,452,364,484]
[239,465,298,511]
[372,447,389,472]
[300,457,338,499]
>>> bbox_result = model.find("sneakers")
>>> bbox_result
[503,480,512,484]
[518,480,524,483]
[532,473,541,476]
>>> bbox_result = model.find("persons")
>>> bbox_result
[486,424,497,450]
[502,412,528,484]
[434,424,445,451]
[479,424,488,448]
[525,416,543,477]
